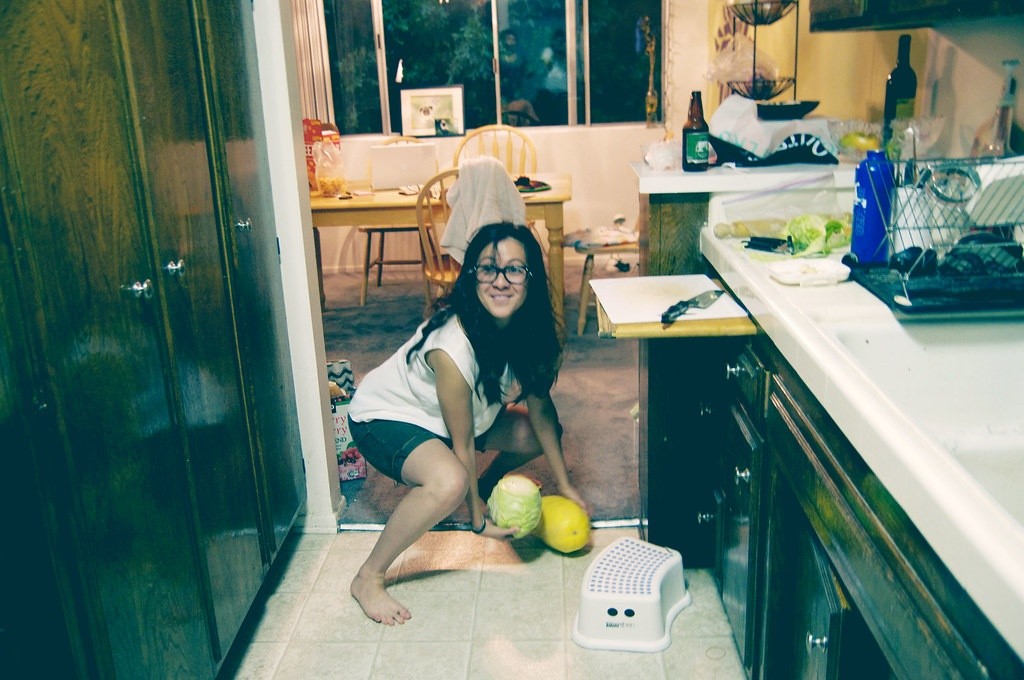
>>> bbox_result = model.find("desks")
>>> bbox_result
[309,173,573,364]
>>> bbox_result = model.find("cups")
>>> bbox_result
[891,188,931,255]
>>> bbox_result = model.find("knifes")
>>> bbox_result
[662,290,727,322]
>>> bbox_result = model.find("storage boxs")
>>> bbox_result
[302,118,340,191]
[573,537,692,653]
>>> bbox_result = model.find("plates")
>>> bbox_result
[769,257,852,288]
[517,180,551,191]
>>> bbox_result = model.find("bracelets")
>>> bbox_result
[471,513,486,535]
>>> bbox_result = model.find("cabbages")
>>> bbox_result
[488,476,543,538]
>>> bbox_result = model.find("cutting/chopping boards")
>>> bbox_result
[588,273,747,324]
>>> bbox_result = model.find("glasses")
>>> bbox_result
[473,264,533,285]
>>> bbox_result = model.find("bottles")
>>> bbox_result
[683,91,708,171]
[851,149,893,265]
[884,34,917,161]
[970,61,1024,159]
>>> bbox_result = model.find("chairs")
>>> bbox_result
[359,137,439,305]
[575,130,674,336]
[416,166,462,322]
[453,124,537,230]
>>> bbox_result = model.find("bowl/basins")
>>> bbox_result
[827,118,945,163]
[757,101,819,120]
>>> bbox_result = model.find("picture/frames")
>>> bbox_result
[398,84,466,139]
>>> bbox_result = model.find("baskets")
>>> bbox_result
[573,534,691,652]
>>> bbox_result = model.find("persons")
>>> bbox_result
[496,31,581,125]
[345,220,590,624]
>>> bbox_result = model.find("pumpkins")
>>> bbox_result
[532,496,592,551]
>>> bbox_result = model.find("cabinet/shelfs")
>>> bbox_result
[0,0,268,680]
[199,0,307,577]
[698,336,896,680]
[727,0,799,103]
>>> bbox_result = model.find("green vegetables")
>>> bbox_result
[786,215,851,256]
